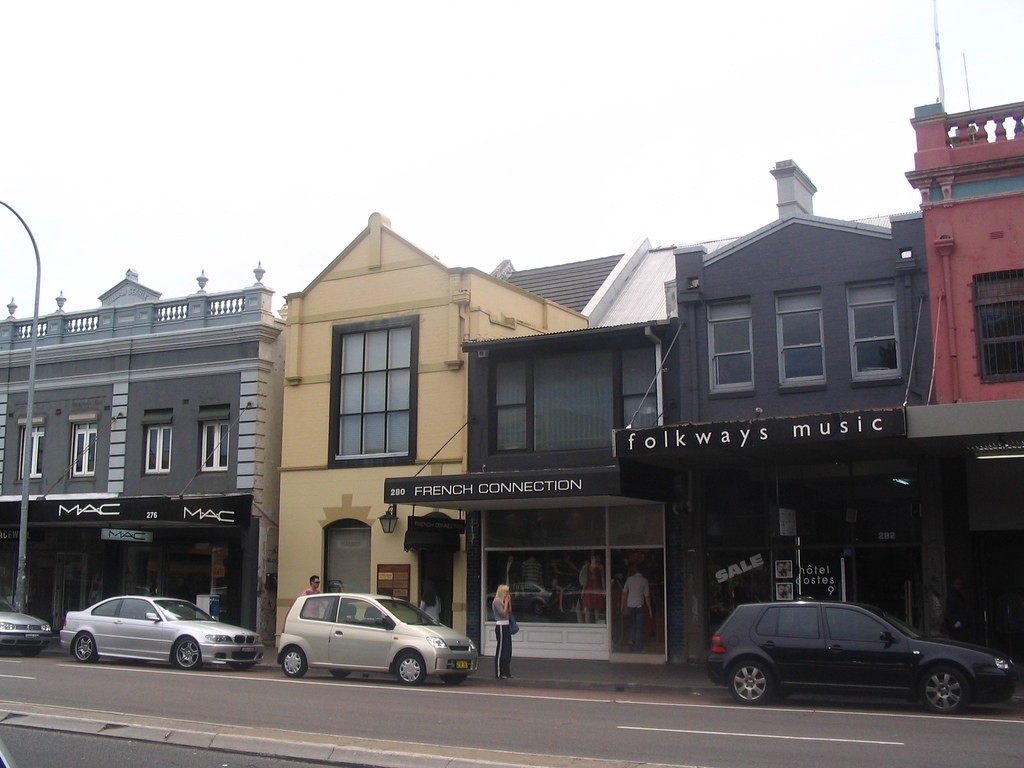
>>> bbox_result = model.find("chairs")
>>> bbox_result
[343,604,359,622]
[363,608,383,624]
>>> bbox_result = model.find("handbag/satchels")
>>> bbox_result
[508,614,519,634]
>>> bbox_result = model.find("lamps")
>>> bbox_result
[378,503,399,533]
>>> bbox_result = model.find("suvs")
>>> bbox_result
[706,597,1016,717]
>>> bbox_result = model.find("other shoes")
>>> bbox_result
[634,647,642,653]
[628,640,634,650]
[495,675,513,679]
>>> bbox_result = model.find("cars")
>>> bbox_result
[58,594,265,671]
[278,592,480,687]
[0,594,54,656]
[487,581,553,617]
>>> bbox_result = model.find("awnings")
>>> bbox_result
[404,512,460,555]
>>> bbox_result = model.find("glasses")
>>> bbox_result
[313,581,320,584]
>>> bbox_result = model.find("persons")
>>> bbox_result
[576,550,605,623]
[500,555,543,622]
[89,571,101,607]
[303,576,329,618]
[778,584,792,600]
[420,589,442,623]
[776,561,792,576]
[492,585,514,680]
[550,552,580,614]
[621,565,652,652]
